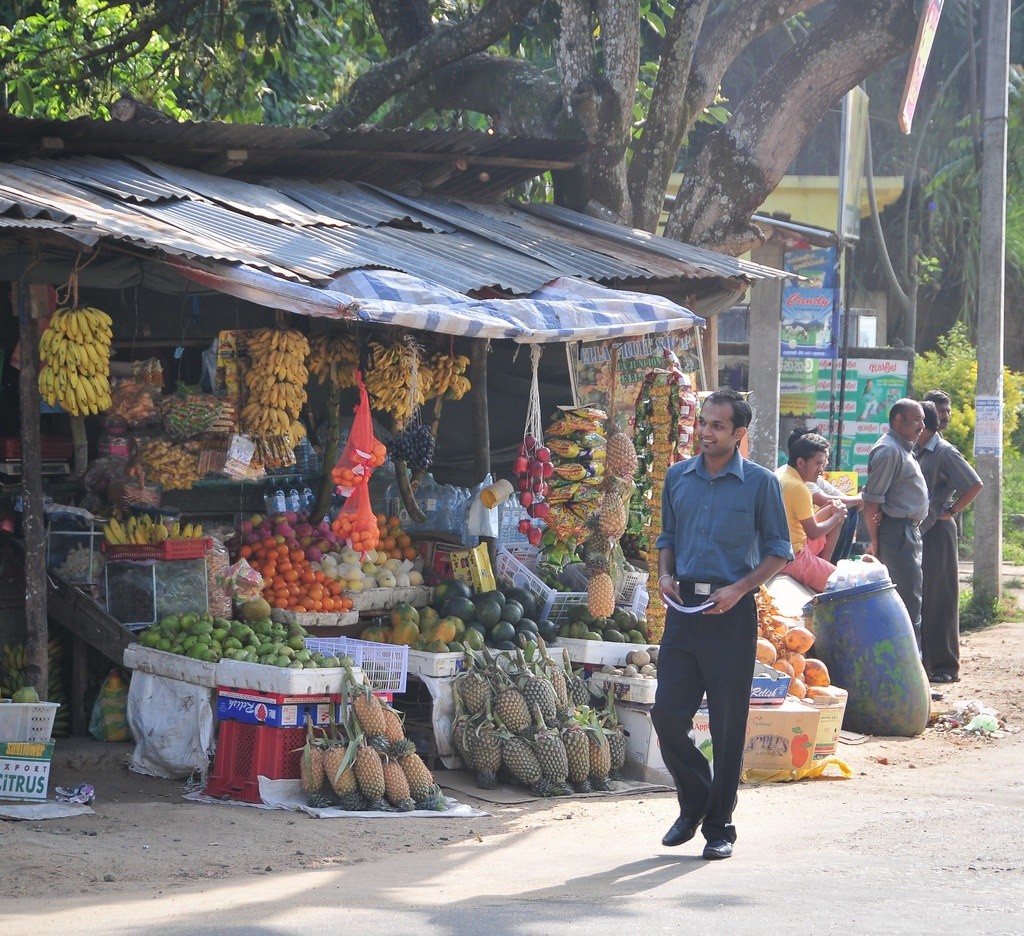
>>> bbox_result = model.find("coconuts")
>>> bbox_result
[11,686,41,702]
[755,627,831,699]
[558,604,651,644]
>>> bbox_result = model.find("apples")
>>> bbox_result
[239,508,344,559]
[320,547,422,589]
[514,434,554,543]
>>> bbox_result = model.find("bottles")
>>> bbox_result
[263,478,286,515]
[370,465,546,546]
[281,477,301,512]
[294,475,314,509]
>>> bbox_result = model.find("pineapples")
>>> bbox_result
[300,687,444,811]
[454,657,626,796]
[583,410,638,620]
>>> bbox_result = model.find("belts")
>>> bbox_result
[905,519,923,528]
[678,580,763,598]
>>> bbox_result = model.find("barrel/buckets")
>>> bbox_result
[812,577,933,737]
[802,600,815,659]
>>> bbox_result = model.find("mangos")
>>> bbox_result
[134,613,353,669]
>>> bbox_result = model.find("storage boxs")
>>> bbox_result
[408,646,565,679]
[496,542,651,627]
[593,659,853,791]
[216,658,368,697]
[0,697,63,802]
[201,688,395,807]
[409,540,496,595]
[555,636,660,669]
[120,642,215,689]
[570,661,606,680]
[304,634,410,695]
[270,607,360,628]
[345,585,429,611]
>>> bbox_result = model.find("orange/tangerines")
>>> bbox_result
[372,513,417,561]
[331,444,386,551]
[239,538,354,613]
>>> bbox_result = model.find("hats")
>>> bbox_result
[787,427,817,445]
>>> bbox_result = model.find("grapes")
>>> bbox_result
[388,425,436,472]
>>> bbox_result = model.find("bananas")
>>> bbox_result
[103,512,202,544]
[37,304,113,418]
[241,324,471,448]
[0,636,72,737]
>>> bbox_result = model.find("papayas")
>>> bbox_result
[361,600,484,654]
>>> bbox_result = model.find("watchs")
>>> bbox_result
[947,507,959,518]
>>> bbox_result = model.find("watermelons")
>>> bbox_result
[434,579,556,652]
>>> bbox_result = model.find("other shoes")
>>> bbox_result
[929,673,960,682]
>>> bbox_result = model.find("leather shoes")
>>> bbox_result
[703,839,733,857]
[662,798,705,847]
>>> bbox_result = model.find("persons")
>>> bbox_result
[776,391,984,681]
[651,389,798,857]
